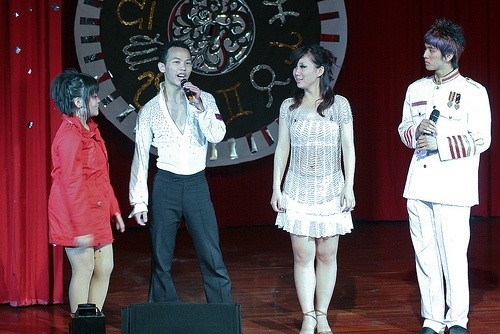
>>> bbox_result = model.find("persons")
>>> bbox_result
[270,46,355,334]
[48,68,125,313]
[128,41,232,306]
[398,20,492,334]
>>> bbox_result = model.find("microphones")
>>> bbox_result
[181,78,193,103]
[424,110,440,135]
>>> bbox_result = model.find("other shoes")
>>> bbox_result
[315,309,333,334]
[447,325,468,334]
[421,327,436,334]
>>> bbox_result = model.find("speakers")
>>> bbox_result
[121,302,242,334]
